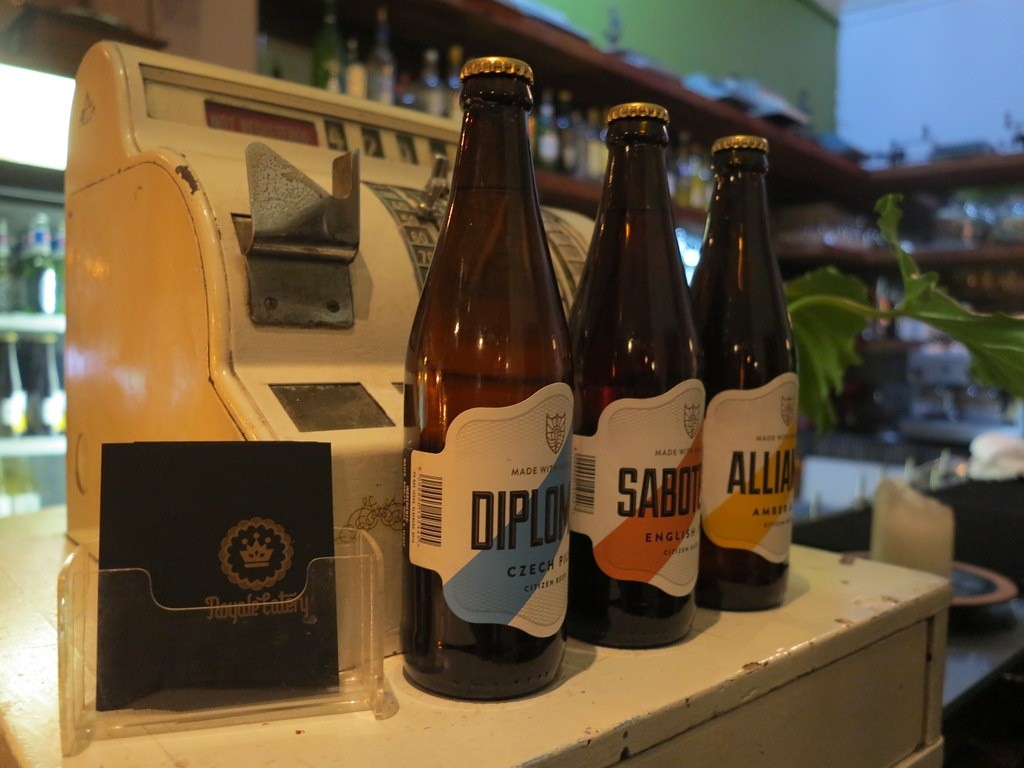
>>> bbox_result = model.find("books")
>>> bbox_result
[96,439,338,713]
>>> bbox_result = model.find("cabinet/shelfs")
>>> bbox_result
[0,1,1024,767]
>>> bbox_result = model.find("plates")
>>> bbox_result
[947,563,1017,609]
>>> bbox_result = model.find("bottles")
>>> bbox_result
[259,1,712,211]
[691,135,800,612]
[0,212,69,439]
[566,102,706,649]
